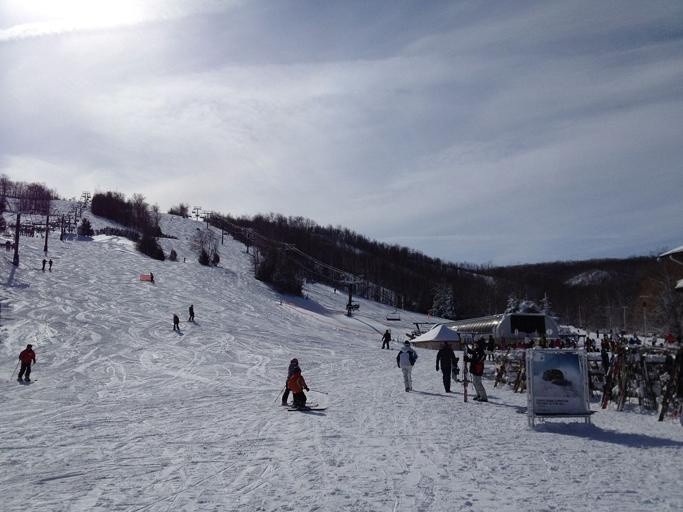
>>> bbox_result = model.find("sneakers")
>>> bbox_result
[17,377,31,382]
[472,395,487,401]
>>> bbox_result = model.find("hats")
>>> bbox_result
[403,340,410,345]
[474,339,482,347]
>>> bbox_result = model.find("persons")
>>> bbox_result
[281,358,310,411]
[16,344,37,382]
[397,339,418,393]
[41,259,54,272]
[149,271,156,283]
[436,328,683,425]
[183,257,186,263]
[171,314,181,331]
[188,304,195,322]
[380,329,391,349]
[346,302,360,312]
